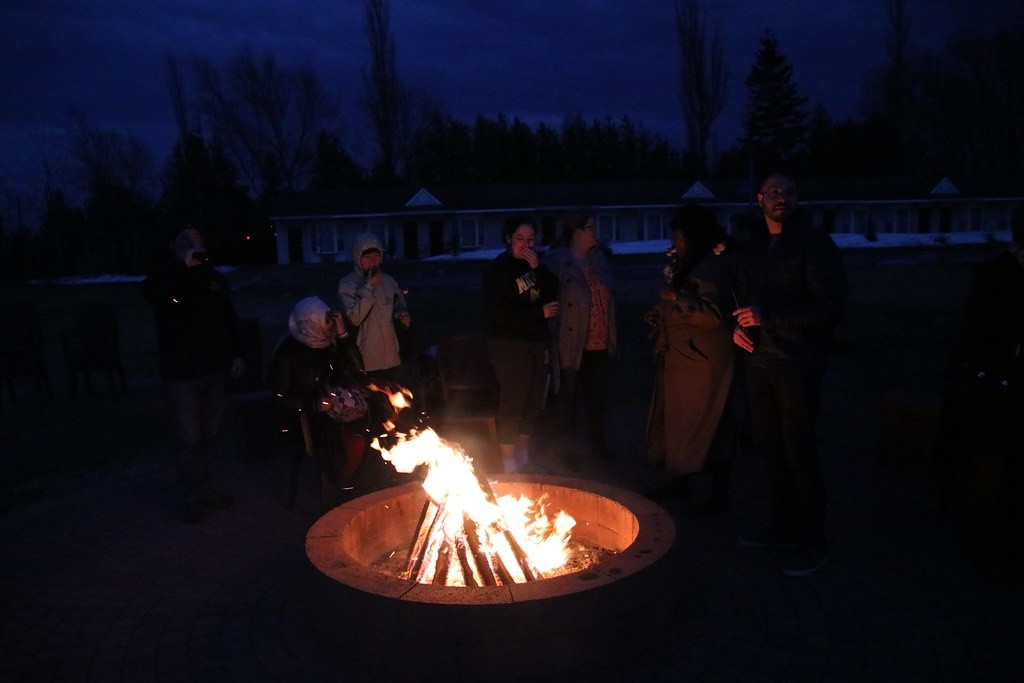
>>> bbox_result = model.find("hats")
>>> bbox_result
[169,221,201,242]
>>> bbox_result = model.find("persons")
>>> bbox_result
[152,218,246,524]
[542,208,620,466]
[335,231,411,424]
[484,215,560,476]
[646,173,852,579]
[269,295,369,522]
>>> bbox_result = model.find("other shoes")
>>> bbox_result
[592,444,618,464]
[769,529,827,557]
[179,497,208,525]
[203,488,231,508]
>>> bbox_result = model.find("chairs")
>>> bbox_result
[0,287,130,407]
[261,360,383,513]
[434,329,497,421]
[346,323,428,426]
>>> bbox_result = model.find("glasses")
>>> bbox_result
[584,224,596,232]
[763,189,794,201]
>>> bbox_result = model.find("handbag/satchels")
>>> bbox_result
[313,370,369,425]
